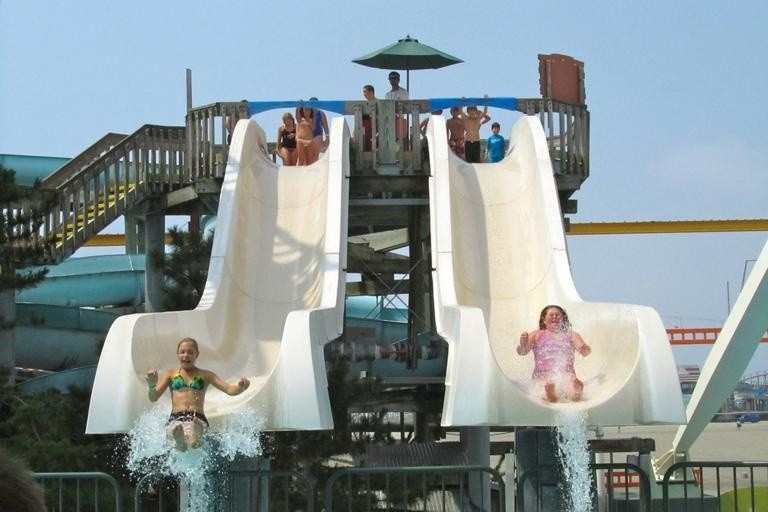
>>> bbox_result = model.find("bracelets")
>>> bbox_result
[148,385,156,392]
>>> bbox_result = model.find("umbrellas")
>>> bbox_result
[351,32,465,95]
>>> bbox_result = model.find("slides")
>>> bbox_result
[422,114,687,427]
[84,114,352,434]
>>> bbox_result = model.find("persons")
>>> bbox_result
[383,71,408,152]
[275,84,504,166]
[145,336,250,452]
[516,304,591,402]
[226,99,247,144]
